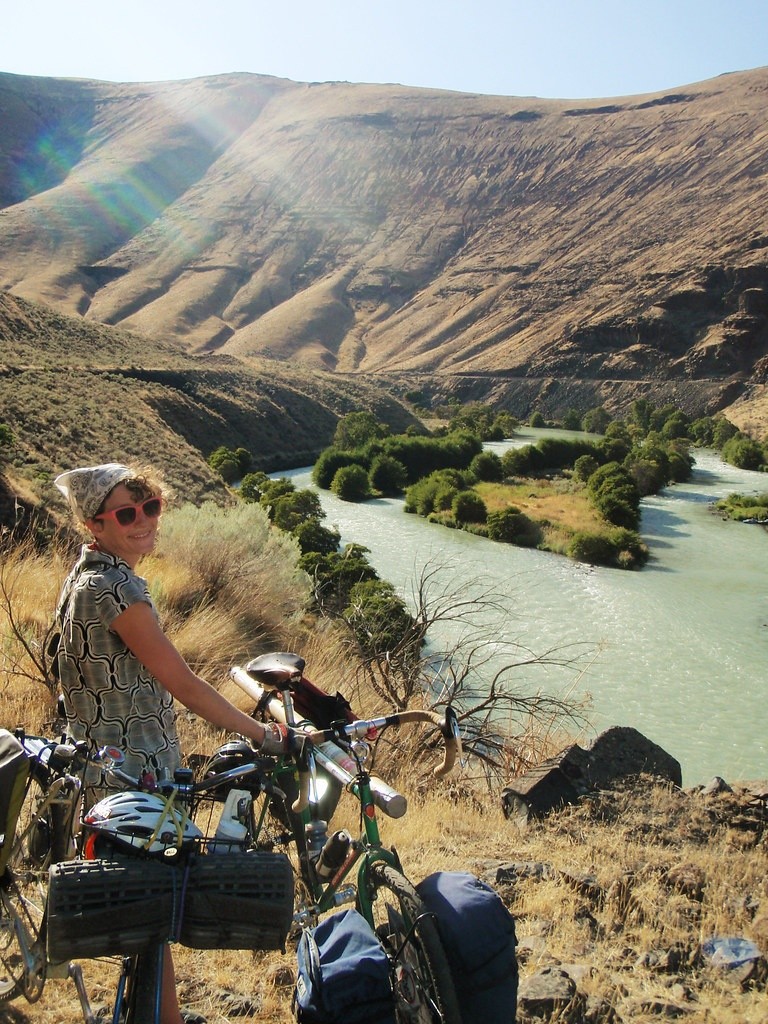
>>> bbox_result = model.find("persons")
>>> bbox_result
[59,465,314,1024]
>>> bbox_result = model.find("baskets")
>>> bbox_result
[79,786,253,862]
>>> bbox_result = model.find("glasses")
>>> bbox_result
[94,496,162,529]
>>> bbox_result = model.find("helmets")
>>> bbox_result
[84,791,204,857]
[202,738,262,802]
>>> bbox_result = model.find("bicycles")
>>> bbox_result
[0,651,467,1023]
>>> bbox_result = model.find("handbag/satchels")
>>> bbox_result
[412,872,518,1023]
[0,729,29,876]
[292,909,395,1024]
